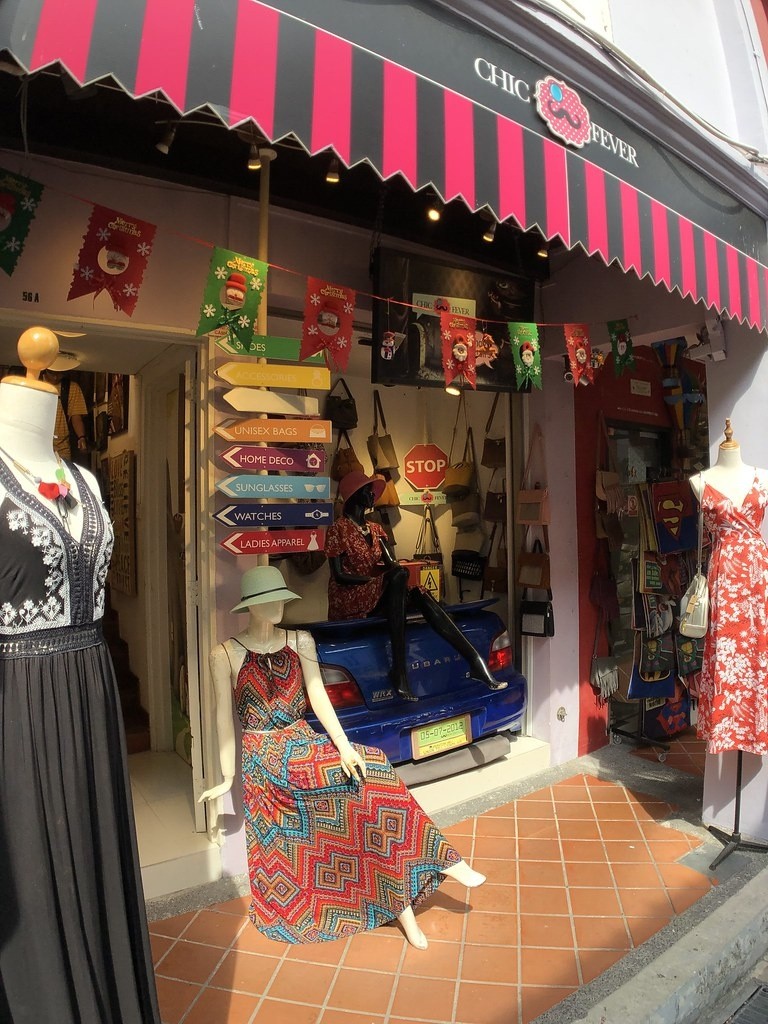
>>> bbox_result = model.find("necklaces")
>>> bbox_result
[346,512,370,536]
[0,446,78,509]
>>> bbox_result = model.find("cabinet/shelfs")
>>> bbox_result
[612,479,710,761]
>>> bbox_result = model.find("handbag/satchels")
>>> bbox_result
[517,586,555,638]
[323,377,359,429]
[368,389,399,470]
[514,424,551,526]
[288,388,328,576]
[587,362,709,743]
[371,470,400,508]
[412,506,446,599]
[332,430,366,481]
[444,388,509,594]
[515,524,553,590]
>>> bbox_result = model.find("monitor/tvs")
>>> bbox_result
[403,252,535,394]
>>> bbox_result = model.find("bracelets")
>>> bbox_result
[79,436,87,440]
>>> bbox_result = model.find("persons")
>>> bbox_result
[0,375,161,1024]
[324,470,509,702]
[38,352,89,462]
[198,565,486,951]
[688,441,768,756]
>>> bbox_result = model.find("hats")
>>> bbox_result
[340,471,386,514]
[227,565,303,614]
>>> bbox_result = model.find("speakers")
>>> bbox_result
[370,247,404,385]
